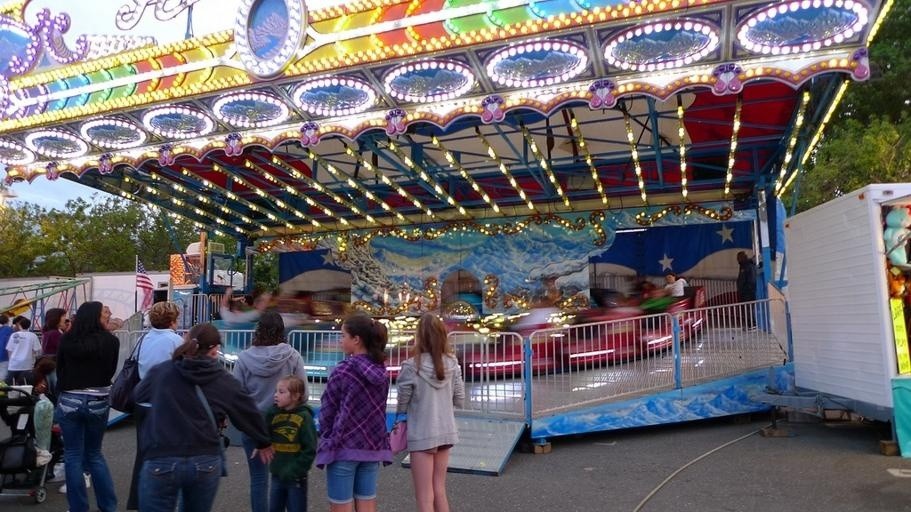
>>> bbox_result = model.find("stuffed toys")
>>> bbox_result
[884,206,911,267]
[888,261,911,306]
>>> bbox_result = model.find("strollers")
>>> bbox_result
[0,386,65,503]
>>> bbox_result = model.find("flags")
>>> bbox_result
[136,258,154,311]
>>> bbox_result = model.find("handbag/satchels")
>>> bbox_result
[109,332,148,412]
[219,434,230,477]
[387,411,407,455]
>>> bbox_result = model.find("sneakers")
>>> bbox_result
[84,472,92,489]
[59,484,67,493]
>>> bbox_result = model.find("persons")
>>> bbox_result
[735,251,760,330]
[532,270,691,323]
